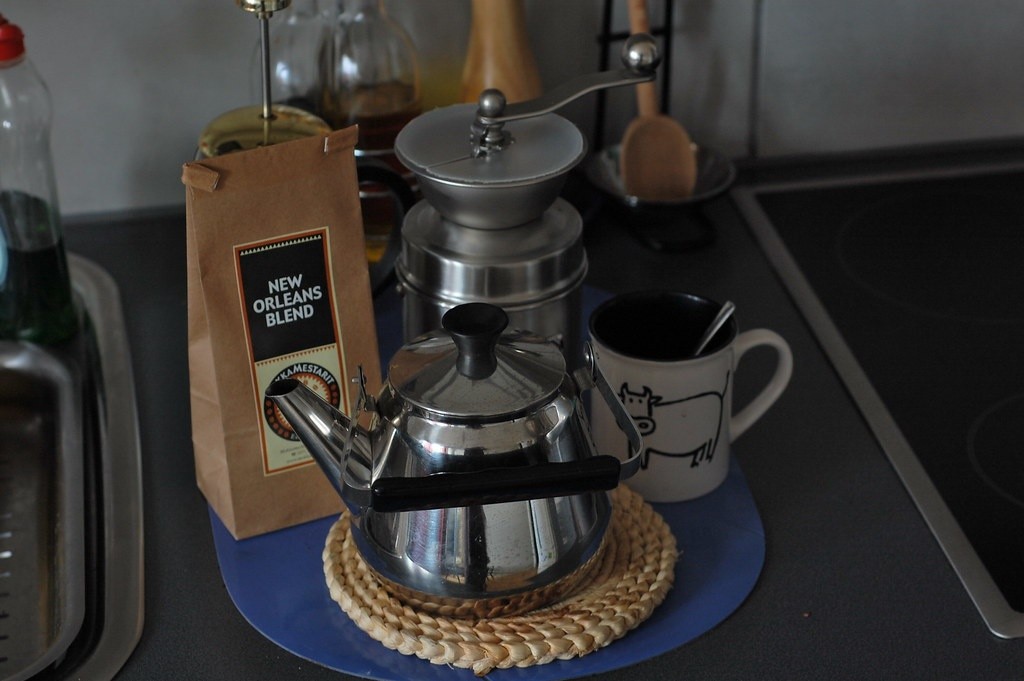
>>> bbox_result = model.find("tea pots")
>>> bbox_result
[264,302,646,622]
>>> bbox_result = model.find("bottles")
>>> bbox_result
[0,22,81,349]
[393,34,663,375]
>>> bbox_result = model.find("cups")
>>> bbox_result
[580,287,794,503]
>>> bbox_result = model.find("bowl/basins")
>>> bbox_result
[590,140,735,222]
